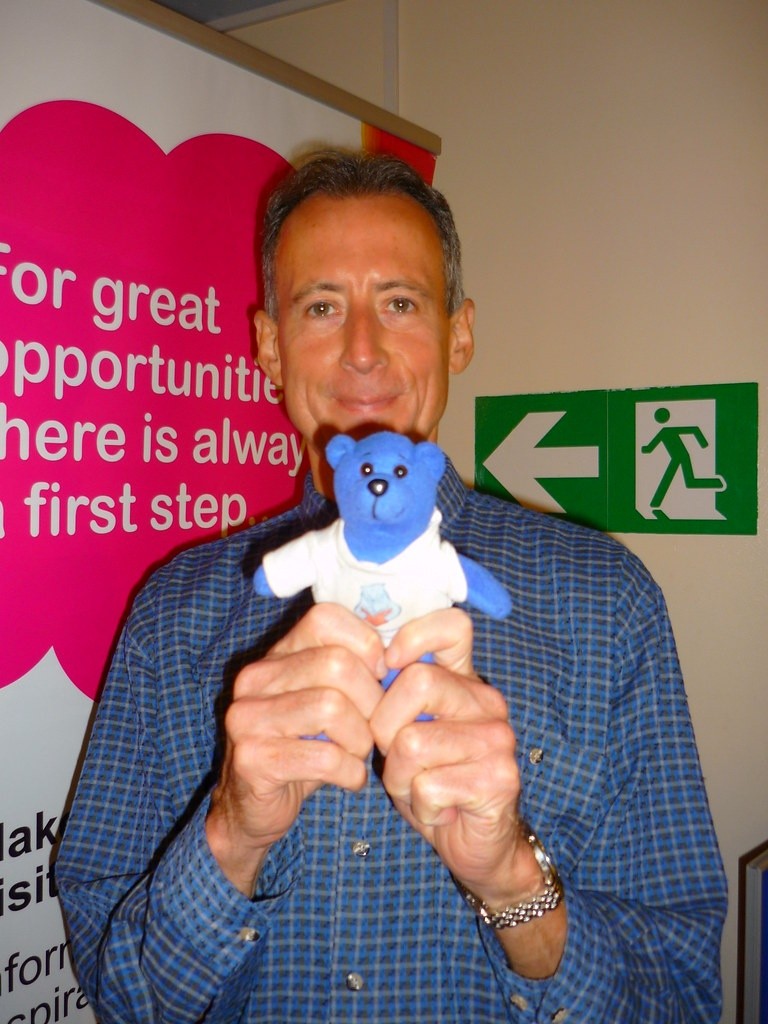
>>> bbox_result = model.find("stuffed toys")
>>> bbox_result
[252,430,513,742]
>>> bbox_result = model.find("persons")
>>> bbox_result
[54,152,731,1024]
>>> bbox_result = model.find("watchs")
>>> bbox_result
[460,822,564,930]
